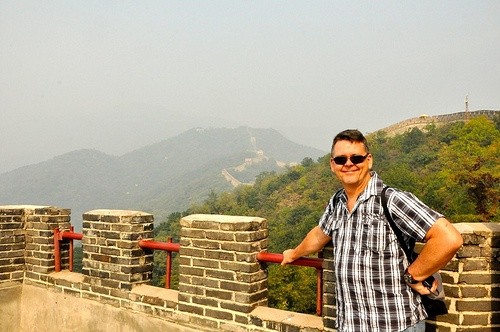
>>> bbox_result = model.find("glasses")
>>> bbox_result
[331,153,369,164]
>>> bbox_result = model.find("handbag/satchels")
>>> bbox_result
[410,251,448,316]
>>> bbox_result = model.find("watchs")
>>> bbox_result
[402,267,419,284]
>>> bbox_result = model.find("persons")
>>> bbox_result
[281,129,462,332]
